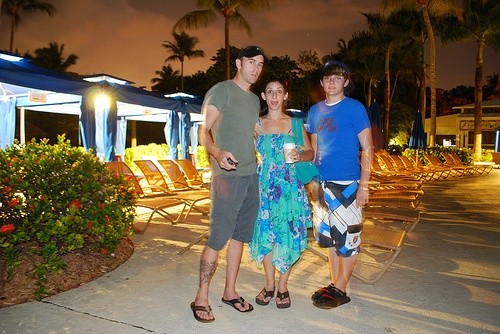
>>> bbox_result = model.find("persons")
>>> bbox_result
[253,75,315,309]
[190,44,261,323]
[306,60,374,309]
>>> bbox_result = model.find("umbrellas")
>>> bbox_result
[367,97,379,122]
[408,109,426,165]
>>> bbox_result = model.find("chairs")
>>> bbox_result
[102,150,500,285]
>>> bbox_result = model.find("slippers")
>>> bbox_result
[255,285,276,306]
[221,296,254,313]
[190,302,215,323]
[277,289,291,308]
[311,287,351,310]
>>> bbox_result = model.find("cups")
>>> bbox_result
[283,142,295,163]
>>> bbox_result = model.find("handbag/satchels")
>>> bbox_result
[291,116,319,185]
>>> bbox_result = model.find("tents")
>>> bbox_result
[0,49,204,163]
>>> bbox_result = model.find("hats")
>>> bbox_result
[237,45,265,58]
[319,59,350,76]
